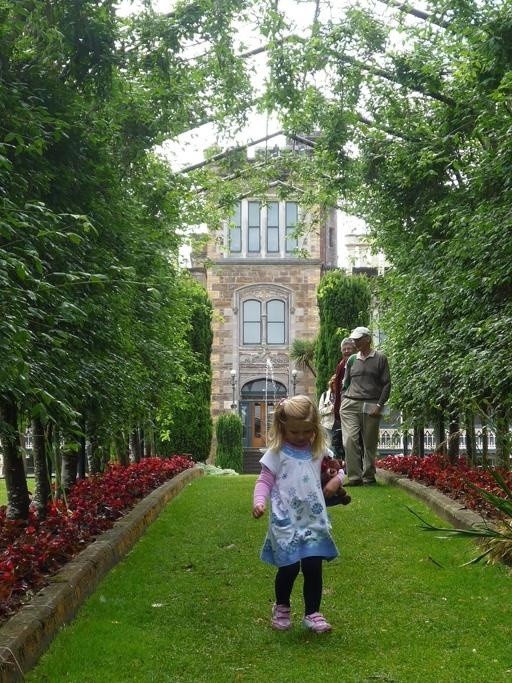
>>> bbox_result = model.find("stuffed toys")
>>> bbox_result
[321,456,351,507]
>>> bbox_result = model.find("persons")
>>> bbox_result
[252,394,345,634]
[316,326,391,486]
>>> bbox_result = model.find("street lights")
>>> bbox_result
[230,369,238,413]
[291,369,301,398]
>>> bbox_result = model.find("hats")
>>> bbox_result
[350,326,371,339]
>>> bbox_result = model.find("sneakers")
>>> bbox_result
[344,480,363,487]
[304,613,331,633]
[365,481,376,485]
[272,602,291,630]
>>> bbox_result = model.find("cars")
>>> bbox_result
[2,446,56,474]
